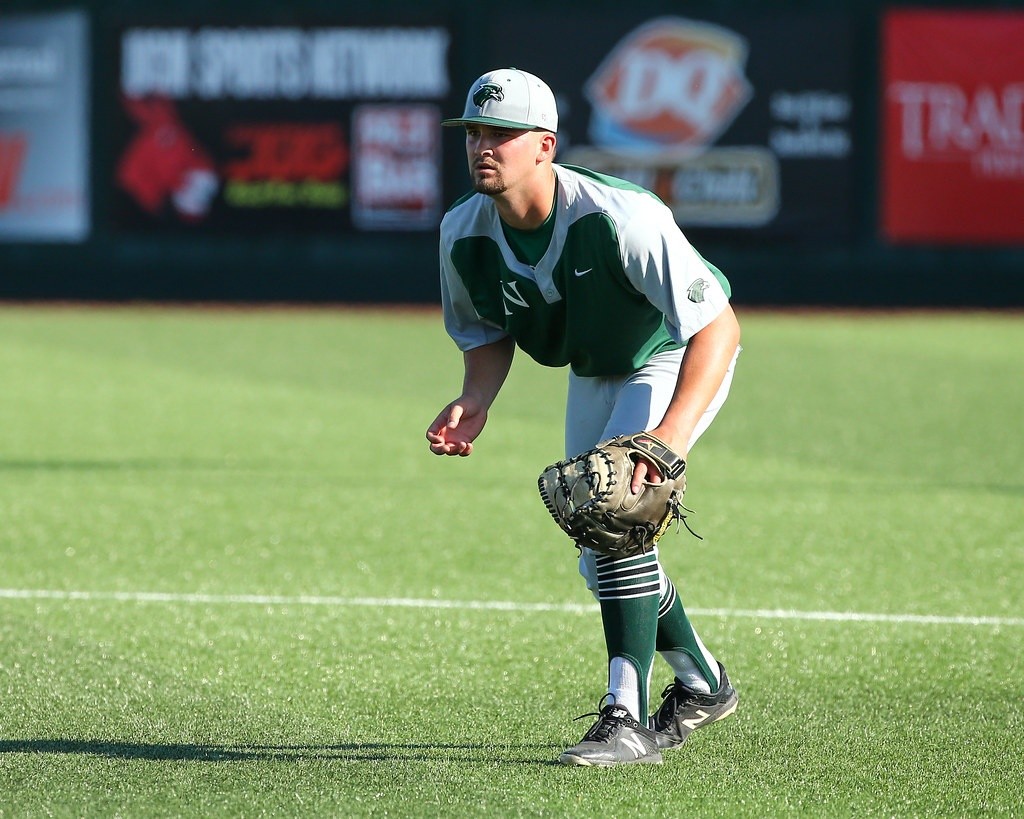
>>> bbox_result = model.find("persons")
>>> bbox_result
[426,67,746,765]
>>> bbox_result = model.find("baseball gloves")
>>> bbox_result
[535,430,687,560]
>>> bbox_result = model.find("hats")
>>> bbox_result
[440,67,558,133]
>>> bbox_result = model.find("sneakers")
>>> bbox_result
[560,693,662,766]
[652,661,739,751]
